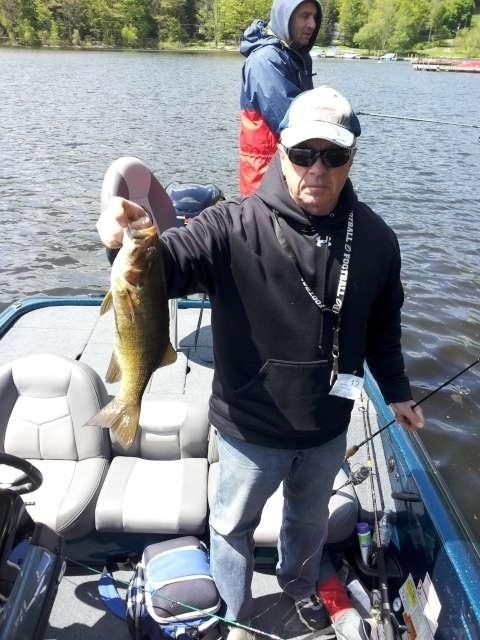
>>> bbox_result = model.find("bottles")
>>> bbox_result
[358,522,373,564]
[372,506,393,547]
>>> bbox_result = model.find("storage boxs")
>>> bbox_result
[141,535,225,640]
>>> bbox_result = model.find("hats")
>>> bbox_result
[279,86,361,147]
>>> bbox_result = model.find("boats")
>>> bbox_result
[0,180,479,640]
[306,45,398,62]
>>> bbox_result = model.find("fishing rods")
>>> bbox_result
[354,110,480,128]
[332,391,408,640]
[64,556,283,640]
[345,356,480,462]
[195,292,206,350]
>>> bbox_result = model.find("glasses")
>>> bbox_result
[285,146,350,168]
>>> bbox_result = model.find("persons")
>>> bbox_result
[89,84,429,640]
[237,0,324,198]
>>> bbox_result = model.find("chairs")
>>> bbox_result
[204,423,357,551]
[93,414,212,540]
[0,354,108,544]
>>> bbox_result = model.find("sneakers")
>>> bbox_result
[294,591,334,631]
